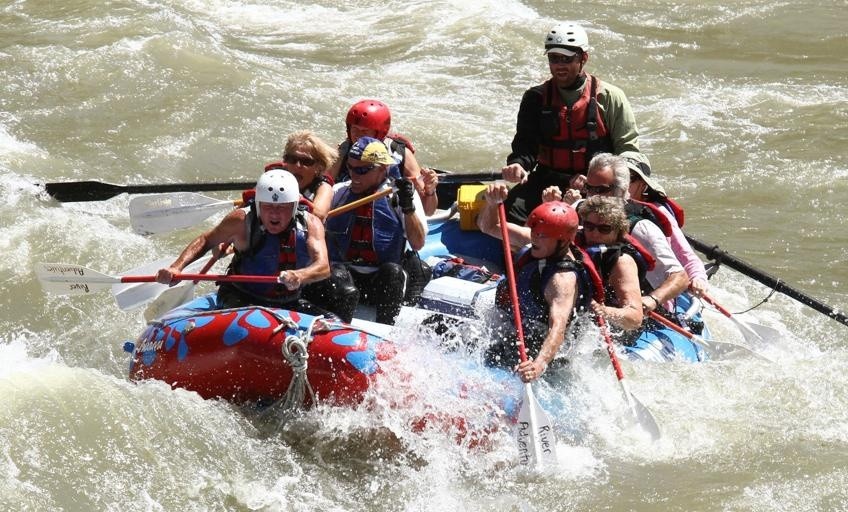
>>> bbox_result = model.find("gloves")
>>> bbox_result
[394,178,416,214]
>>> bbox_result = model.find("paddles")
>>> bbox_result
[596,315,660,445]
[109,188,396,324]
[647,285,783,366]
[495,199,557,474]
[33,264,281,293]
[33,170,524,202]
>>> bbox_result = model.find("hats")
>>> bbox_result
[543,47,577,57]
[347,136,397,165]
[618,151,667,198]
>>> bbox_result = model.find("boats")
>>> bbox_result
[120,214,715,455]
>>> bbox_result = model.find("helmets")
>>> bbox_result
[545,21,589,53]
[527,200,579,241]
[254,169,299,220]
[345,100,390,142]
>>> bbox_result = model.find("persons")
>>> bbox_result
[502,22,639,226]
[476,21,712,383]
[541,150,707,346]
[155,169,343,322]
[478,182,579,384]
[279,99,439,325]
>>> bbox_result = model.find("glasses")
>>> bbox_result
[283,154,318,167]
[548,54,574,63]
[344,162,374,175]
[581,221,617,234]
[584,183,616,195]
[630,173,639,183]
